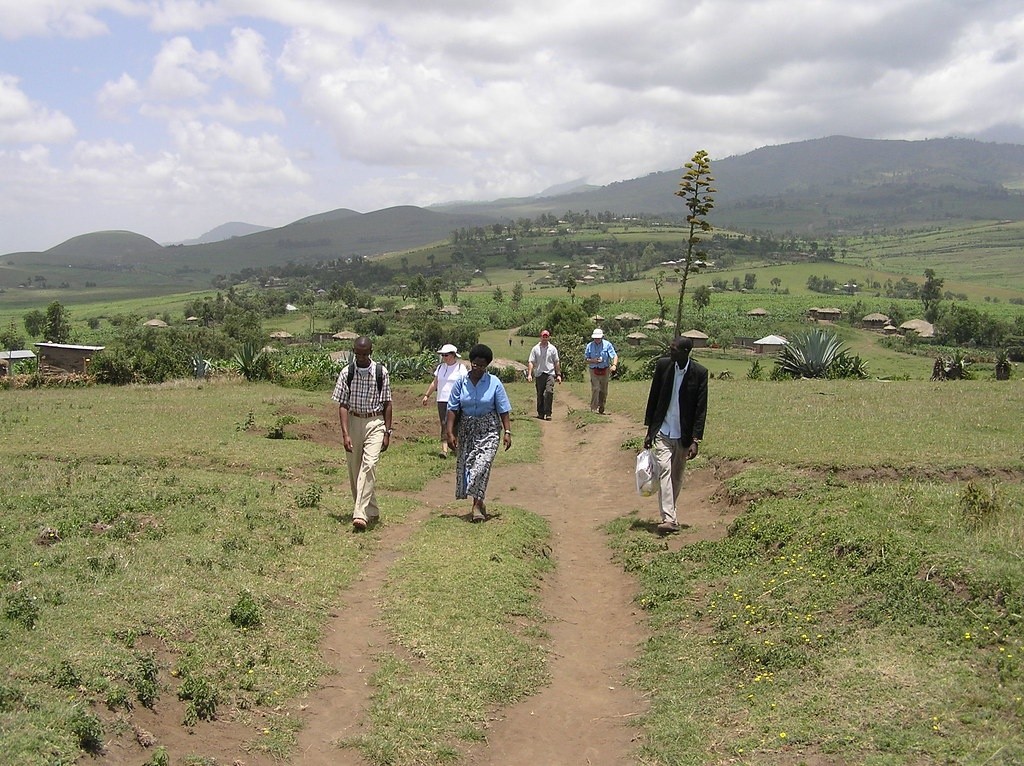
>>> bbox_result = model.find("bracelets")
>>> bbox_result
[384,430,392,436]
[613,364,617,367]
[556,374,561,377]
[425,395,429,397]
[506,429,511,435]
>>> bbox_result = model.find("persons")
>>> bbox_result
[644,337,708,532]
[509,337,512,346]
[528,330,561,420]
[447,344,512,523]
[422,344,468,458]
[331,336,392,530]
[521,338,524,346]
[585,328,618,414]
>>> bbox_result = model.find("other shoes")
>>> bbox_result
[353,518,367,530]
[658,521,680,533]
[468,504,487,522]
[367,516,379,524]
[438,451,447,459]
[591,408,599,414]
[539,412,545,419]
[546,414,551,420]
[599,411,606,415]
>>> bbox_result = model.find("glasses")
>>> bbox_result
[471,361,487,371]
[441,354,452,357]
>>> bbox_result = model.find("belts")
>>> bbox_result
[349,411,383,418]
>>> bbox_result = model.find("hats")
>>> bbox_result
[541,330,550,337]
[436,344,457,354]
[590,328,603,339]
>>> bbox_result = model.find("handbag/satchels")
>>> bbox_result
[593,367,607,375]
[635,447,660,496]
